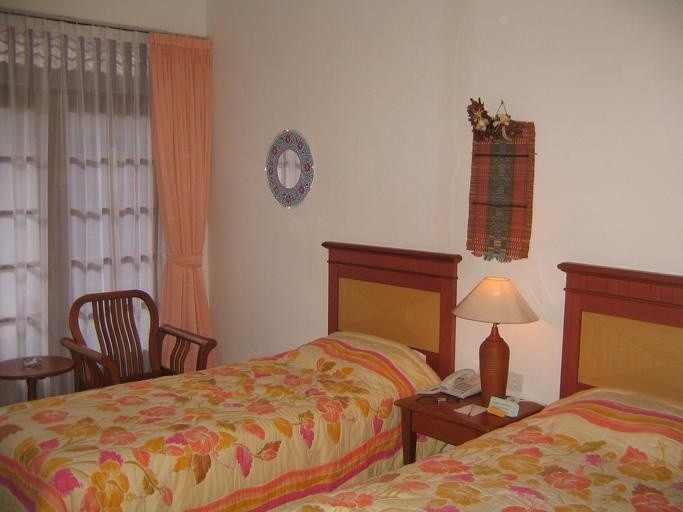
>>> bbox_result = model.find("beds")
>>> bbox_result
[256,262,683,512]
[0,241,463,512]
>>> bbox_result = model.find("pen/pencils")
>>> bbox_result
[467,401,474,417]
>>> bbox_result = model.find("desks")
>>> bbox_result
[394,390,545,465]
[0,355,76,401]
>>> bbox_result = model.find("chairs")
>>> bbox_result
[59,289,217,391]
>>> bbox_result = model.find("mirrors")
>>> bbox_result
[265,129,315,208]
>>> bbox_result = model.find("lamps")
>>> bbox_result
[451,276,539,407]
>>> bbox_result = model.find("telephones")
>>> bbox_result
[439,368,481,399]
